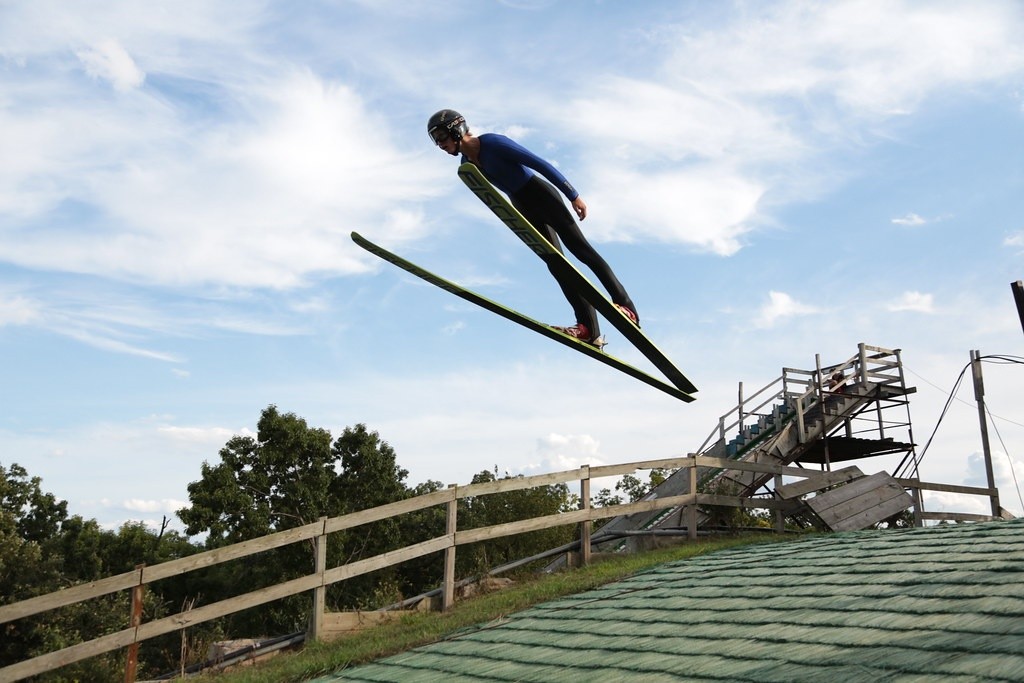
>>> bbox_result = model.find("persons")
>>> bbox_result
[428,109,640,352]
[828,367,847,397]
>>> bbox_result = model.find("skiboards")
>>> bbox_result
[350,161,699,402]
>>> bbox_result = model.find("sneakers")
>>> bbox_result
[611,302,641,329]
[551,324,601,347]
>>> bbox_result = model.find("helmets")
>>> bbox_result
[427,110,469,144]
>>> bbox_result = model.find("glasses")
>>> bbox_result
[432,116,465,144]
